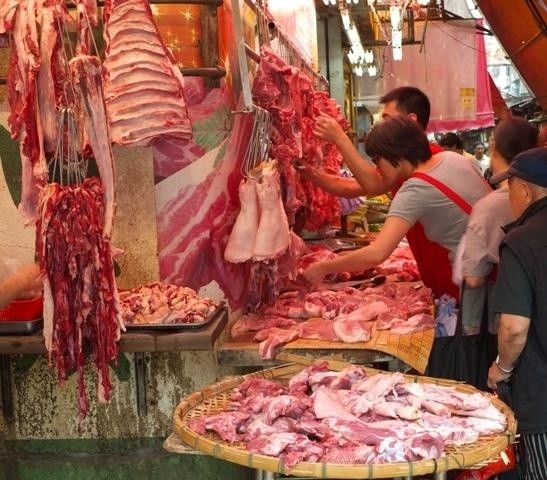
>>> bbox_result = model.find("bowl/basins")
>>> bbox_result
[3,289,42,322]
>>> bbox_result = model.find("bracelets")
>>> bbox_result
[494,354,514,373]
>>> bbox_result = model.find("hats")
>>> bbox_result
[488,146,547,190]
[492,114,540,166]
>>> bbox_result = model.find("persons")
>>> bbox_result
[292,83,457,301]
[472,142,490,173]
[298,112,499,391]
[437,131,475,161]
[484,144,547,480]
[457,116,538,388]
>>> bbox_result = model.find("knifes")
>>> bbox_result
[328,274,387,291]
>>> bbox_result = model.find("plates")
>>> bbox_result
[335,240,355,250]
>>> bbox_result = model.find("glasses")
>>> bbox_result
[370,154,381,166]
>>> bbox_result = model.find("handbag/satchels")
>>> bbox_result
[424,274,500,396]
[456,378,523,480]
